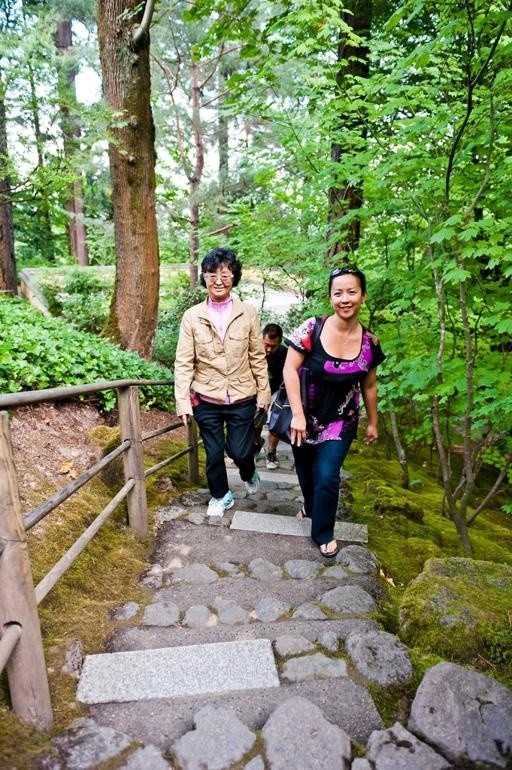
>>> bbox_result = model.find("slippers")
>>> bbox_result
[318,538,339,558]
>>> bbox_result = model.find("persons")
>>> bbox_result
[251,323,288,470]
[174,248,272,519]
[283,266,387,557]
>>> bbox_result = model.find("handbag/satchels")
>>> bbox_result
[265,366,314,446]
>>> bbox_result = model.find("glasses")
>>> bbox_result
[203,272,235,282]
[328,264,360,279]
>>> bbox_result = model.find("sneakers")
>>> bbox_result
[205,490,235,519]
[253,437,266,459]
[295,506,309,519]
[243,456,261,495]
[263,446,280,472]
